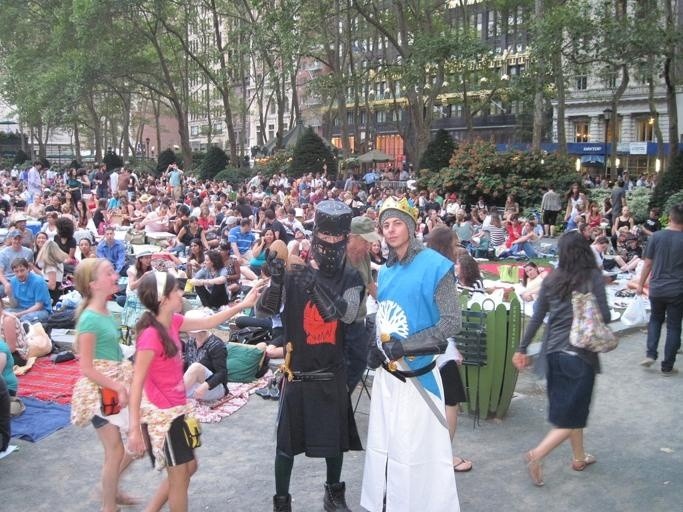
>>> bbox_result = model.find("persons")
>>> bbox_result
[426,223,473,473]
[256,170,315,199]
[186,169,256,249]
[570,215,586,235]
[181,306,228,403]
[633,207,662,242]
[174,237,206,279]
[279,208,303,242]
[255,199,366,511]
[286,226,311,262]
[452,207,474,249]
[635,204,683,375]
[418,189,429,208]
[119,250,160,329]
[614,235,641,281]
[414,214,427,243]
[218,242,241,301]
[540,183,562,238]
[255,208,265,229]
[359,195,463,512]
[589,234,618,284]
[453,253,485,310]
[498,221,542,259]
[427,188,443,209]
[502,194,519,223]
[440,192,460,228]
[609,178,627,236]
[0,159,77,453]
[585,201,602,229]
[579,223,595,245]
[613,169,634,190]
[504,214,523,249]
[635,170,658,191]
[443,192,451,211]
[275,207,286,220]
[190,249,232,313]
[513,262,551,325]
[70,257,138,512]
[426,208,443,233]
[511,230,611,487]
[264,209,289,245]
[368,239,387,285]
[470,195,506,248]
[563,182,590,232]
[610,205,634,251]
[315,163,419,218]
[522,214,544,240]
[239,227,277,281]
[77,162,187,260]
[581,168,609,190]
[126,269,267,512]
[343,216,381,397]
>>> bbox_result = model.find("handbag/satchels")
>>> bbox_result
[21,321,52,359]
[568,273,618,353]
[98,347,120,417]
[150,380,202,450]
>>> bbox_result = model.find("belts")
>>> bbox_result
[379,360,437,383]
[285,367,335,382]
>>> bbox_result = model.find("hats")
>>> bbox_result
[225,216,237,225]
[377,195,423,268]
[9,229,25,239]
[14,214,27,223]
[314,200,353,237]
[136,249,152,258]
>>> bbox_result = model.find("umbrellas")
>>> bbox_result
[355,143,395,172]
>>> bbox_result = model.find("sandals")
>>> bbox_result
[115,490,139,507]
[573,451,596,472]
[453,457,472,472]
[523,449,545,487]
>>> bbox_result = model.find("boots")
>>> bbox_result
[322,481,352,512]
[272,493,292,512]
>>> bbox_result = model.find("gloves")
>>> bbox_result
[260,248,284,285]
[290,262,317,295]
[376,339,403,368]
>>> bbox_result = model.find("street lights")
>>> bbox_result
[146,137,150,161]
[145,137,152,157]
